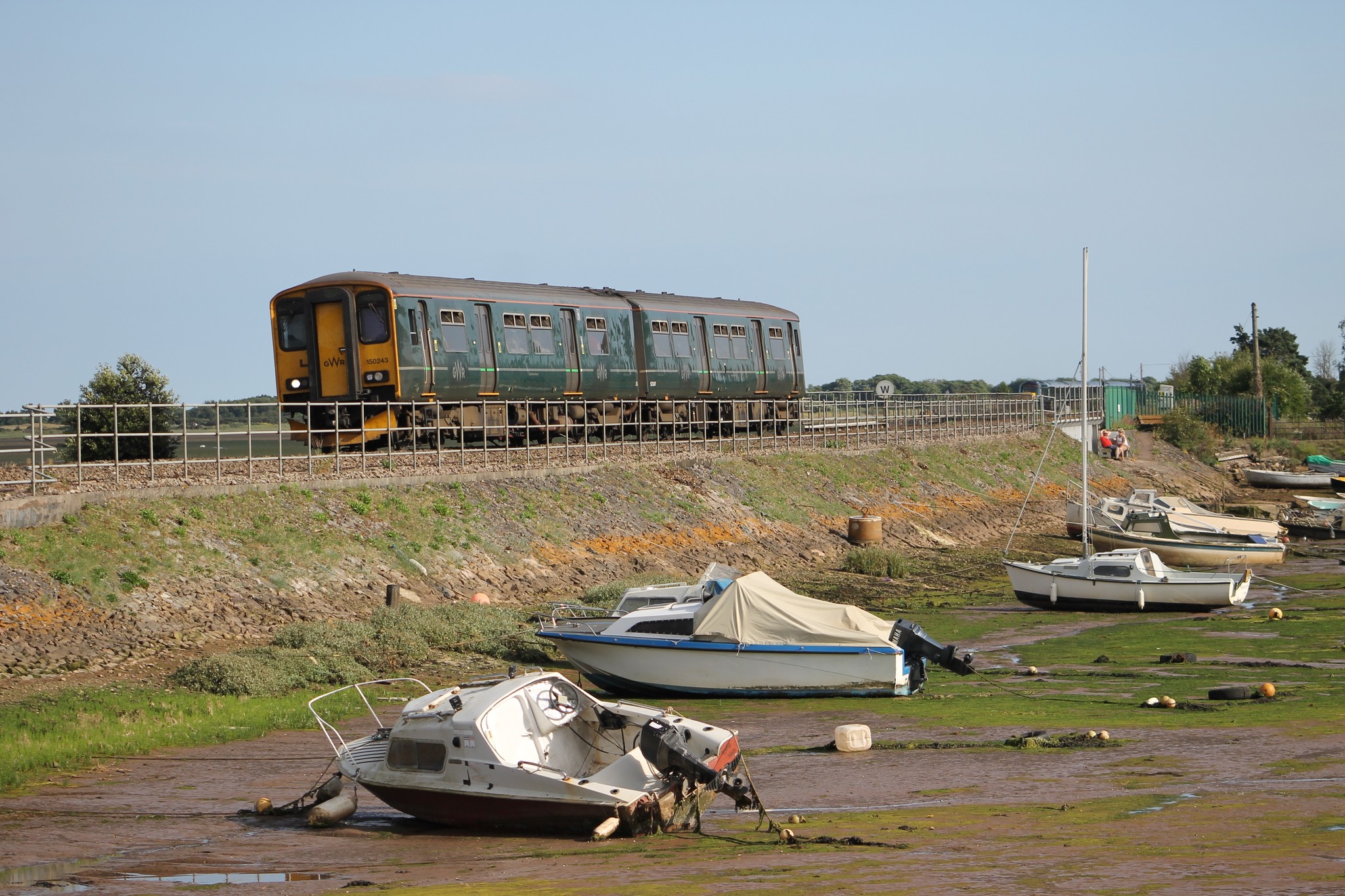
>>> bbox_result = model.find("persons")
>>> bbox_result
[1116,428,1128,461]
[1099,429,1121,461]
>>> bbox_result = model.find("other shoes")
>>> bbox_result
[1114,458,1121,461]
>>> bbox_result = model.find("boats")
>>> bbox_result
[1241,467,1340,490]
[534,560,977,700]
[306,665,754,843]
[1306,455,1345,478]
[1064,475,1345,569]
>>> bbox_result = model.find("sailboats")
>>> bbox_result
[999,247,1256,615]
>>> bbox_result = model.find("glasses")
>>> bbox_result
[1104,433,1107,434]
[1118,431,1123,433]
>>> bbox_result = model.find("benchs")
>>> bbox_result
[1098,438,1130,460]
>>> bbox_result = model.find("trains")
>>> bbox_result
[1019,379,1148,419]
[269,270,807,453]
